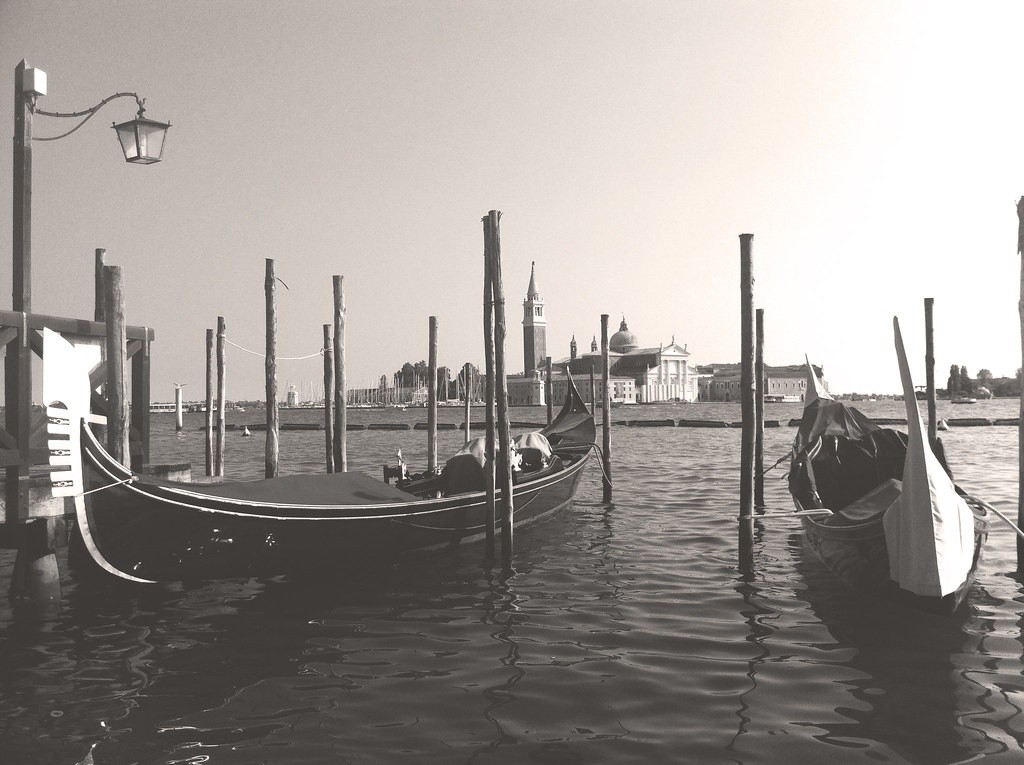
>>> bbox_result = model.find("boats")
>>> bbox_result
[596,400,624,408]
[69,363,597,586]
[960,387,994,399]
[786,317,991,617]
[230,405,245,412]
[951,394,976,403]
[763,395,785,403]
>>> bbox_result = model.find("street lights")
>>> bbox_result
[5,90,170,521]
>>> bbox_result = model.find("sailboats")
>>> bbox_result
[279,370,497,408]
